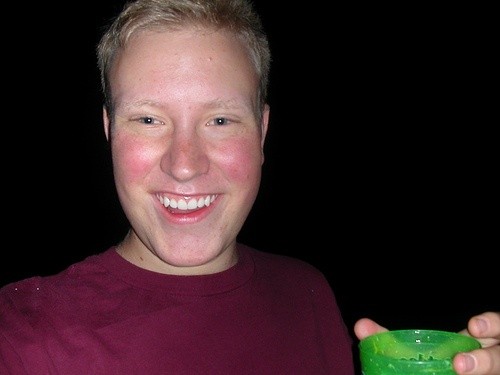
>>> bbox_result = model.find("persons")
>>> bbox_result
[0,0,499,375]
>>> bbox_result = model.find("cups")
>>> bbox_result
[358,329,481,375]
